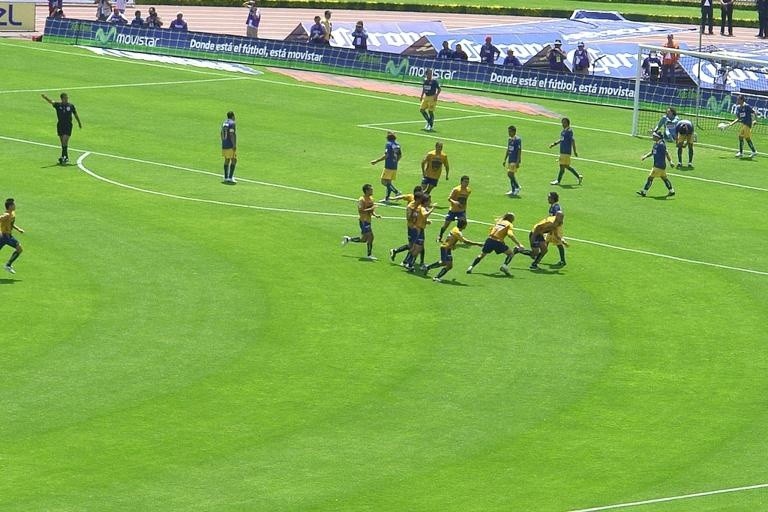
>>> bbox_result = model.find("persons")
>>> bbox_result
[755,1,768,39]
[547,39,567,66]
[728,94,758,158]
[40,92,84,164]
[49,1,64,18]
[342,184,381,259]
[169,13,187,30]
[309,10,334,46]
[503,49,522,66]
[644,34,680,86]
[243,0,261,38]
[549,118,583,185]
[701,1,734,36]
[388,175,569,282]
[572,42,589,74]
[421,141,449,194]
[479,36,500,65]
[96,1,162,28]
[352,21,368,51]
[220,110,240,186]
[636,107,695,199]
[418,70,441,129]
[0,197,27,276]
[438,41,468,60]
[714,59,729,86]
[503,126,522,196]
[372,132,402,204]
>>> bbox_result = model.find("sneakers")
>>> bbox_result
[550,180,560,185]
[578,175,583,185]
[636,191,646,196]
[224,178,238,184]
[506,187,521,196]
[500,264,512,276]
[368,256,378,261]
[390,249,441,282]
[735,151,757,157]
[5,266,15,273]
[342,236,350,246]
[665,191,675,197]
